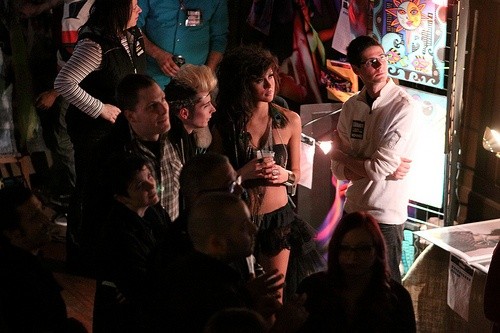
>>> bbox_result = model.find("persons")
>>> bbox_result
[1,0,415,333]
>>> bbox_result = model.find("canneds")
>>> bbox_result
[174,55,185,67]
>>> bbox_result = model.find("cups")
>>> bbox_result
[257,150,275,163]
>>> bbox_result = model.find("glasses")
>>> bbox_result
[194,174,242,194]
[355,53,387,66]
[340,245,375,257]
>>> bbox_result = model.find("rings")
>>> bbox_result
[273,174,279,179]
